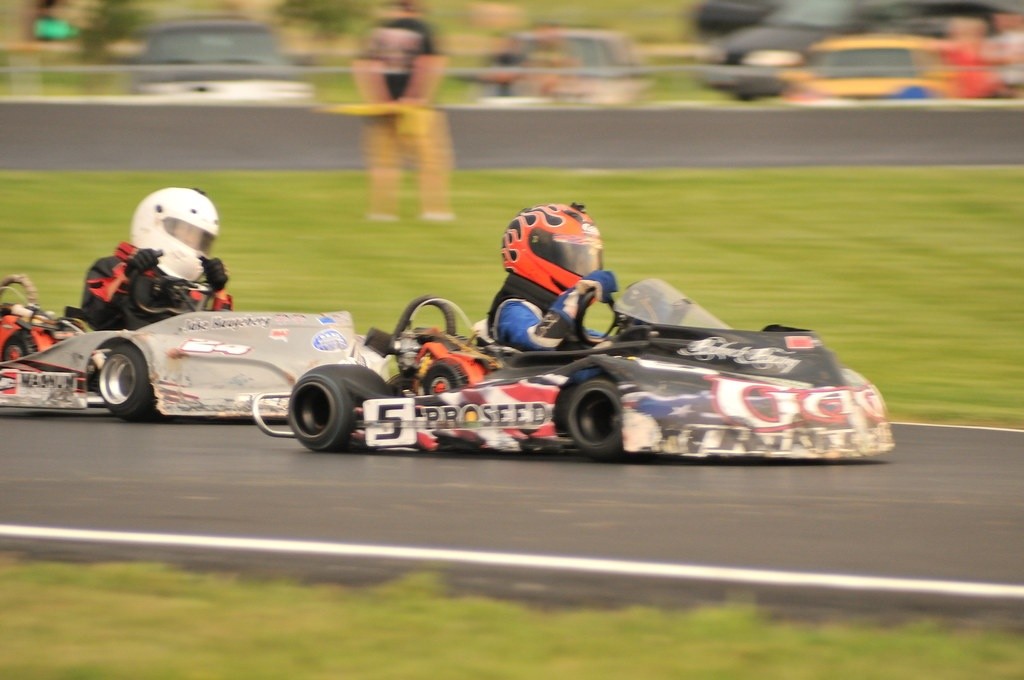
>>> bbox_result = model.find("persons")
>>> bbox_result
[928,9,1024,100]
[80,185,232,391]
[27,0,77,43]
[351,0,454,223]
[487,203,640,368]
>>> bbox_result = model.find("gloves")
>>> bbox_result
[124,247,164,279]
[199,256,228,291]
[547,270,619,332]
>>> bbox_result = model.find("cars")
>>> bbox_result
[775,31,995,99]
[124,20,316,102]
[510,28,643,107]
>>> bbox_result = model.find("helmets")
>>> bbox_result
[129,187,219,281]
[501,203,604,296]
